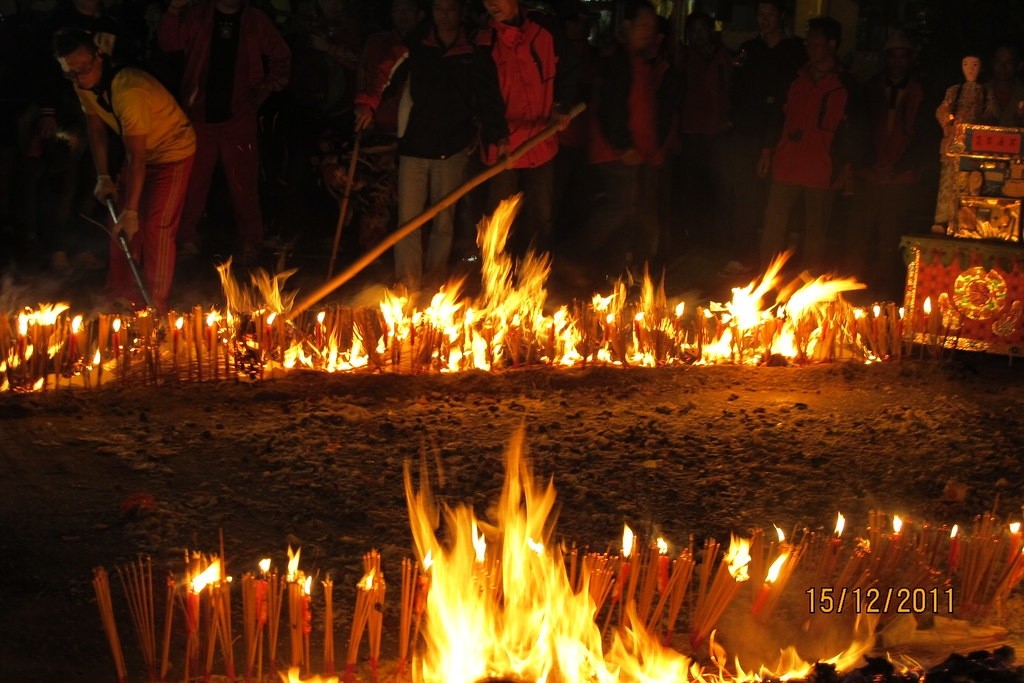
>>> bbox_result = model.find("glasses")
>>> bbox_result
[66,55,95,79]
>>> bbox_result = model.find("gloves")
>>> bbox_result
[113,210,139,241]
[93,175,118,205]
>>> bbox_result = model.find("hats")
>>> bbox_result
[884,30,913,52]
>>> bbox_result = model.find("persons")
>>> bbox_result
[0,0,1024,308]
[53,27,198,313]
[931,52,1001,232]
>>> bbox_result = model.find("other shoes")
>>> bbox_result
[717,260,754,279]
